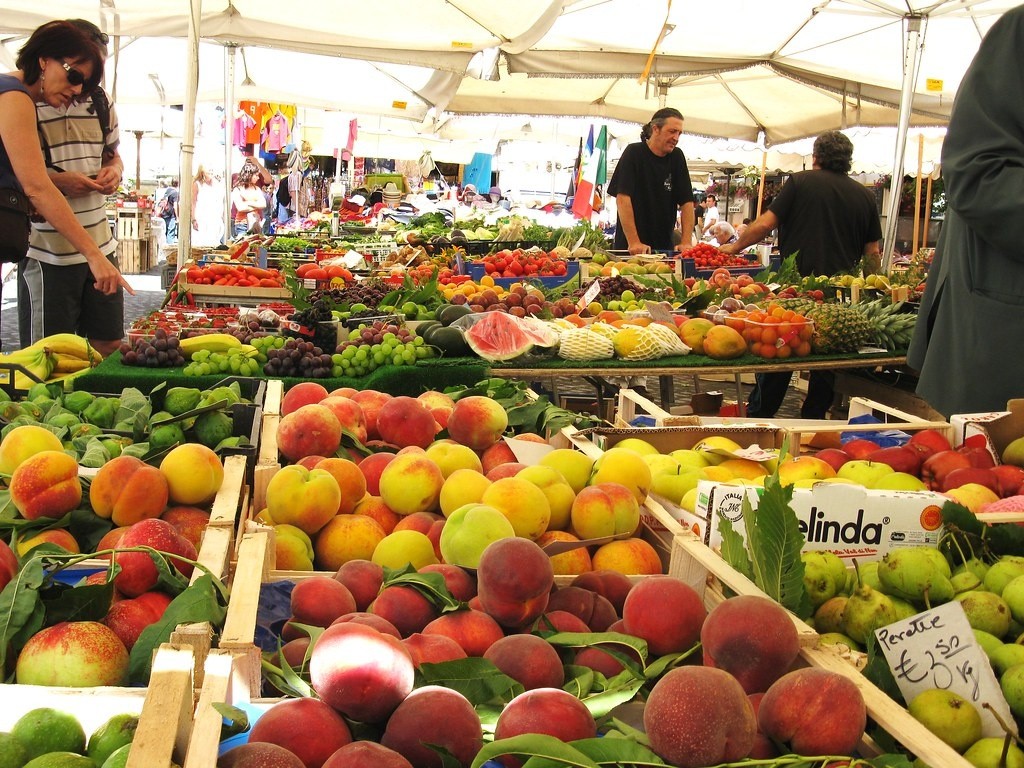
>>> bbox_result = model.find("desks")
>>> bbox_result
[414,350,907,427]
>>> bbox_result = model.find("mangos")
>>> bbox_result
[545,311,747,361]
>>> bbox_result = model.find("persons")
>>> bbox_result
[703,195,720,259]
[713,221,744,256]
[232,156,273,237]
[163,178,179,244]
[719,130,882,416]
[232,162,266,235]
[155,180,168,215]
[0,19,135,359]
[906,5,1023,421]
[601,108,696,401]
[276,156,315,221]
[191,163,227,247]
[737,224,747,237]
[694,196,705,242]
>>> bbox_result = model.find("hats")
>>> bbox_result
[382,183,401,203]
[301,140,312,171]
[354,184,370,196]
[401,194,417,204]
[286,151,299,176]
[436,205,453,217]
[369,192,382,205]
[397,202,413,212]
[464,184,476,195]
[490,187,502,196]
[347,195,366,206]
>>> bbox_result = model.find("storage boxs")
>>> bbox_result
[0,208,1023,768]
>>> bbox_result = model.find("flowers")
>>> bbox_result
[116,179,137,201]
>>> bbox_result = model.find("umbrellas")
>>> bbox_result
[0,0,1023,302]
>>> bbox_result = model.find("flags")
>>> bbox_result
[565,138,582,214]
[572,125,607,221]
[577,124,602,215]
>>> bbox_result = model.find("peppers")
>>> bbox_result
[159,266,196,309]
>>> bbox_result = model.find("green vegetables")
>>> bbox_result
[267,212,611,251]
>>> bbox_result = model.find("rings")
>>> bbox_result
[114,186,117,190]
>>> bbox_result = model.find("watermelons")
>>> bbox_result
[457,312,560,361]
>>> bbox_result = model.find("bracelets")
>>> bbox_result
[257,164,260,166]
[192,219,196,224]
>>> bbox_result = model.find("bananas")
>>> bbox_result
[0,332,103,390]
[178,334,259,358]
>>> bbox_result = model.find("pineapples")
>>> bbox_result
[751,298,921,354]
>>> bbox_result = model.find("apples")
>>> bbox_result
[607,428,1024,520]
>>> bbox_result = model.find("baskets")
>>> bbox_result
[346,242,397,263]
[316,249,372,265]
[160,265,178,288]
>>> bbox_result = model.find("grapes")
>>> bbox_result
[119,321,332,377]
[329,320,436,378]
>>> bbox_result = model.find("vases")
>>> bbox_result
[122,200,136,208]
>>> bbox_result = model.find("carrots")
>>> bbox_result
[186,264,285,286]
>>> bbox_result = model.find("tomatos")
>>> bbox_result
[668,242,759,269]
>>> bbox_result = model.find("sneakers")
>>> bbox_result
[603,384,623,405]
[627,386,654,402]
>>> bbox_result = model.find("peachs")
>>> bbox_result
[0,383,885,768]
[655,268,910,313]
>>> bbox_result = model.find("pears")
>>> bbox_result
[788,543,1024,768]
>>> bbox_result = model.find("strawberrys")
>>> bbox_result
[128,310,236,342]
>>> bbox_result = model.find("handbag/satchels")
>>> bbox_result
[0,188,31,262]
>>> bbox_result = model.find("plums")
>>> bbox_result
[450,286,574,318]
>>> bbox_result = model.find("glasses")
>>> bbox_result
[58,56,91,95]
[83,32,109,45]
